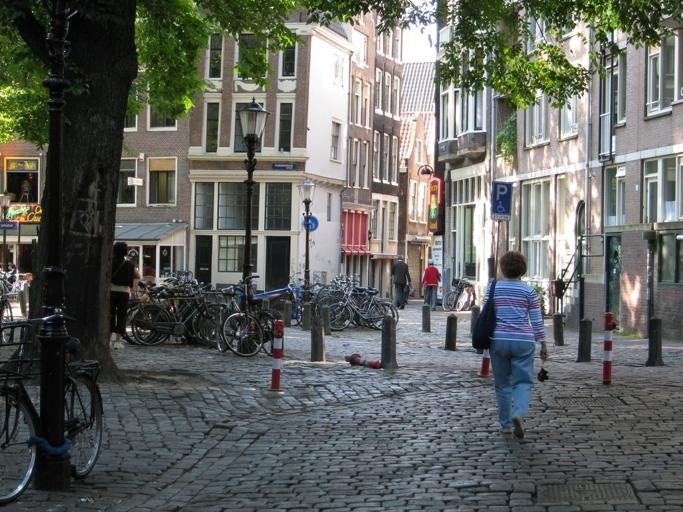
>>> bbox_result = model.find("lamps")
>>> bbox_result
[417,165,434,176]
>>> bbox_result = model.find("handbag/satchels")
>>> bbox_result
[471,279,496,350]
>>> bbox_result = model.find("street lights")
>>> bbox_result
[237,97,270,356]
[298,180,314,300]
[1,191,10,267]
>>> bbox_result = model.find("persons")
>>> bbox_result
[404,284,410,301]
[19,179,29,203]
[391,256,411,309]
[6,262,16,291]
[482,251,548,440]
[109,242,140,350]
[422,260,441,310]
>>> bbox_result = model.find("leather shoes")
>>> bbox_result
[511,415,524,438]
[499,426,512,434]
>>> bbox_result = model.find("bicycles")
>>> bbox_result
[0,306,102,504]
[0,267,14,344]
[276,273,399,331]
[442,278,475,311]
[124,268,285,357]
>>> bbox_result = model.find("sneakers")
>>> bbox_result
[113,342,124,349]
